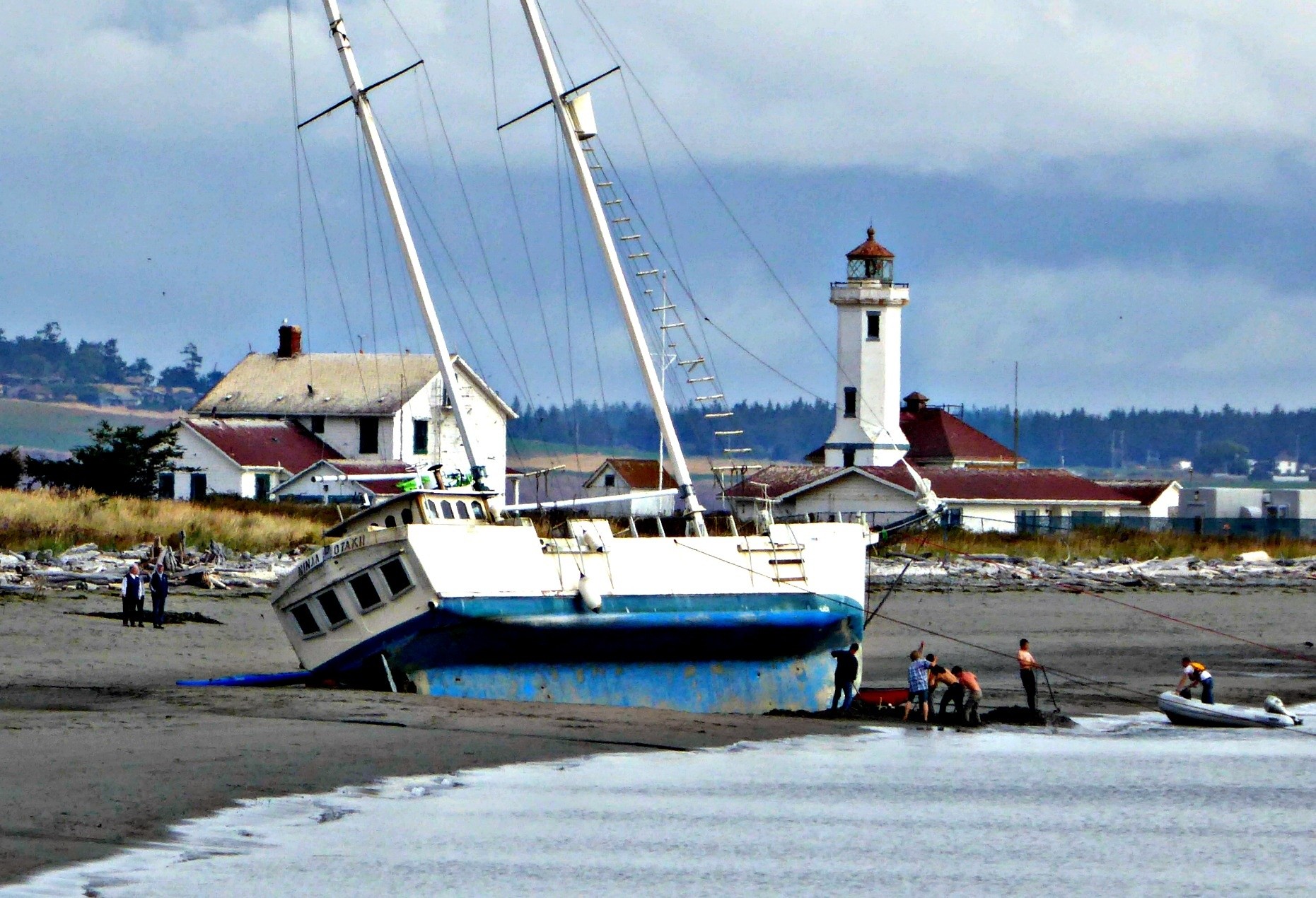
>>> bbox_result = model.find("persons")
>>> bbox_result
[149,563,168,629]
[903,651,984,726]
[1016,638,1043,708]
[122,564,146,628]
[831,642,859,711]
[1176,657,1214,704]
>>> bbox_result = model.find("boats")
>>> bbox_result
[1157,686,1304,727]
[855,686,910,706]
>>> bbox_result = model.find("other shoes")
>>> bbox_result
[123,624,129,627]
[154,625,164,629]
[138,624,144,628]
[130,624,137,627]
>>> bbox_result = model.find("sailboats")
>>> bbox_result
[261,3,953,719]
